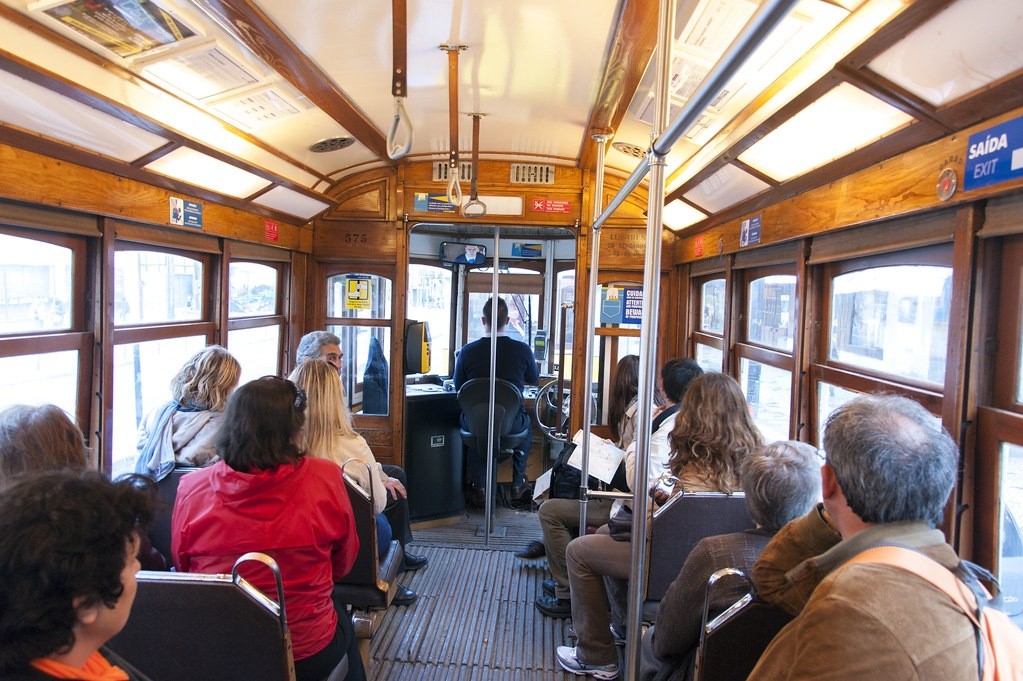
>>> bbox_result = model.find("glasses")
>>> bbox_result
[815,448,829,462]
[259,375,303,408]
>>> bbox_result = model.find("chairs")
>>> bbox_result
[454,375,529,536]
[693,566,787,681]
[604,490,754,632]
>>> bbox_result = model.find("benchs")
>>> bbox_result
[109,552,349,681]
[138,455,404,621]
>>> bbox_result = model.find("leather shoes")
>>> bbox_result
[391,582,417,605]
[398,550,427,574]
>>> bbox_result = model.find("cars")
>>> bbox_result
[998,500,1023,631]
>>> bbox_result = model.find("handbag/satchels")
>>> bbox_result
[608,509,633,542]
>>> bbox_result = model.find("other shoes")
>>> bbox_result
[609,624,622,640]
[569,626,578,639]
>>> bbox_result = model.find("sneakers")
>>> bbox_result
[514,541,546,561]
[534,596,573,621]
[513,474,529,499]
[542,580,557,597]
[471,479,485,496]
[556,646,620,680]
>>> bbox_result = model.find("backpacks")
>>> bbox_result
[548,445,599,501]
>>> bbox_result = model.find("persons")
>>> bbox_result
[171,377,368,681]
[553,372,765,681]
[0,469,154,681]
[286,358,392,556]
[456,245,485,264]
[453,295,539,499]
[639,439,825,681]
[514,355,666,560]
[296,332,428,604]
[136,344,240,468]
[0,405,167,572]
[742,396,991,681]
[535,357,703,617]
